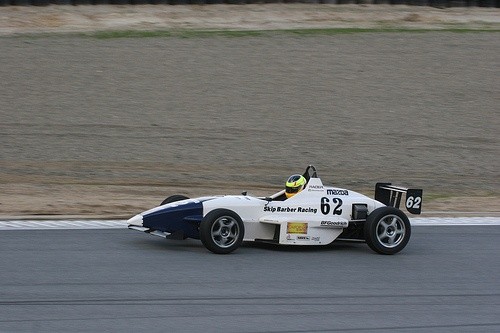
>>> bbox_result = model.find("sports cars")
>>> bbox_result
[126,165,423,256]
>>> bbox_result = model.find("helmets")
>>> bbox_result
[285,173,307,199]
